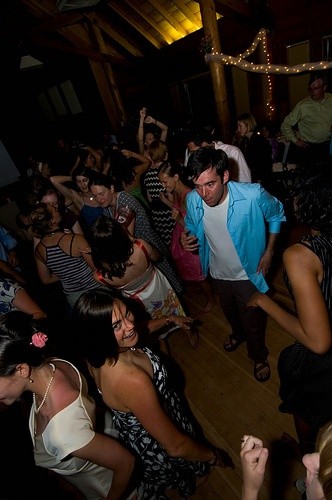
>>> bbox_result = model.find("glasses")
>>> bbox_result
[308,86,323,92]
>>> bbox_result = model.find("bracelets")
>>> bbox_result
[155,120,157,125]
[165,316,169,325]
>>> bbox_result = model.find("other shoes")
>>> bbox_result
[295,451,310,464]
[293,478,306,494]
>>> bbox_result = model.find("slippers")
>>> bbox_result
[223,334,241,352]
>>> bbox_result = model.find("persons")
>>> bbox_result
[0,73,332,500]
[32,363,56,415]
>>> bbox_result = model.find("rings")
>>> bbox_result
[240,440,247,443]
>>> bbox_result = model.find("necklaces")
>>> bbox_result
[85,193,94,201]
[119,348,136,353]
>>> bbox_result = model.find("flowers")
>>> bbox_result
[32,331,48,348]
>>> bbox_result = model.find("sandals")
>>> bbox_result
[191,331,199,350]
[254,359,270,382]
